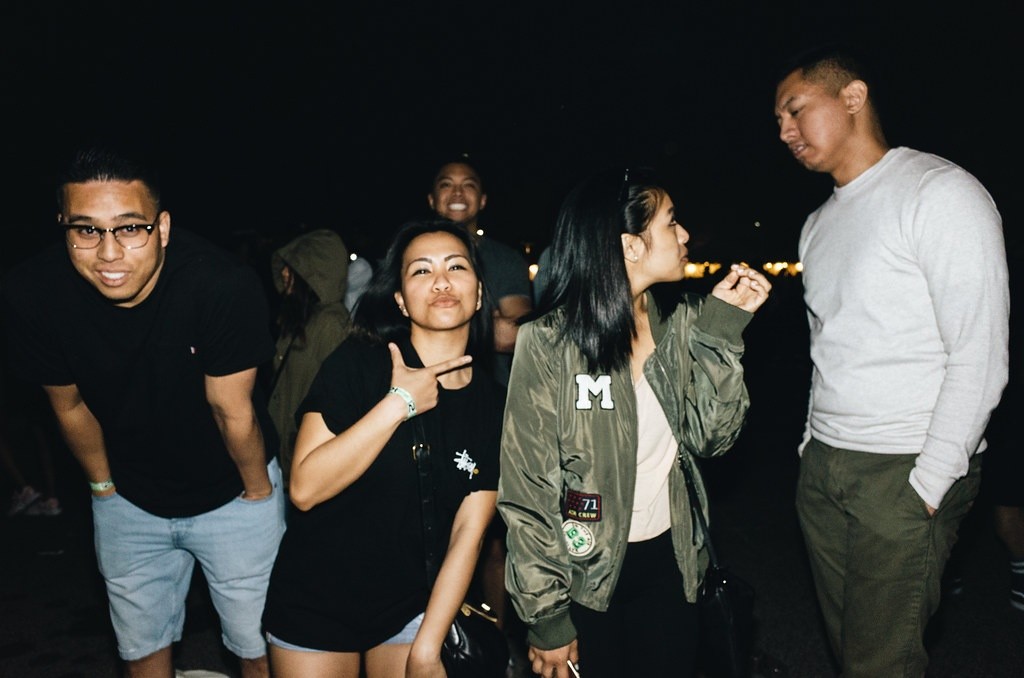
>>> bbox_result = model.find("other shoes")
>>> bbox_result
[1010,588,1024,611]
[948,572,963,594]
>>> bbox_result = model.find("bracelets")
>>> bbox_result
[89,478,114,494]
[390,385,417,419]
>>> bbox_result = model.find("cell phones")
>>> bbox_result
[566,659,580,678]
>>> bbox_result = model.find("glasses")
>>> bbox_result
[59,214,158,249]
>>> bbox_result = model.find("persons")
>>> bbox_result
[27,141,288,678]
[772,48,1012,678]
[264,147,774,678]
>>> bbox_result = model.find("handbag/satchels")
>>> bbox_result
[699,566,758,678]
[440,600,510,678]
